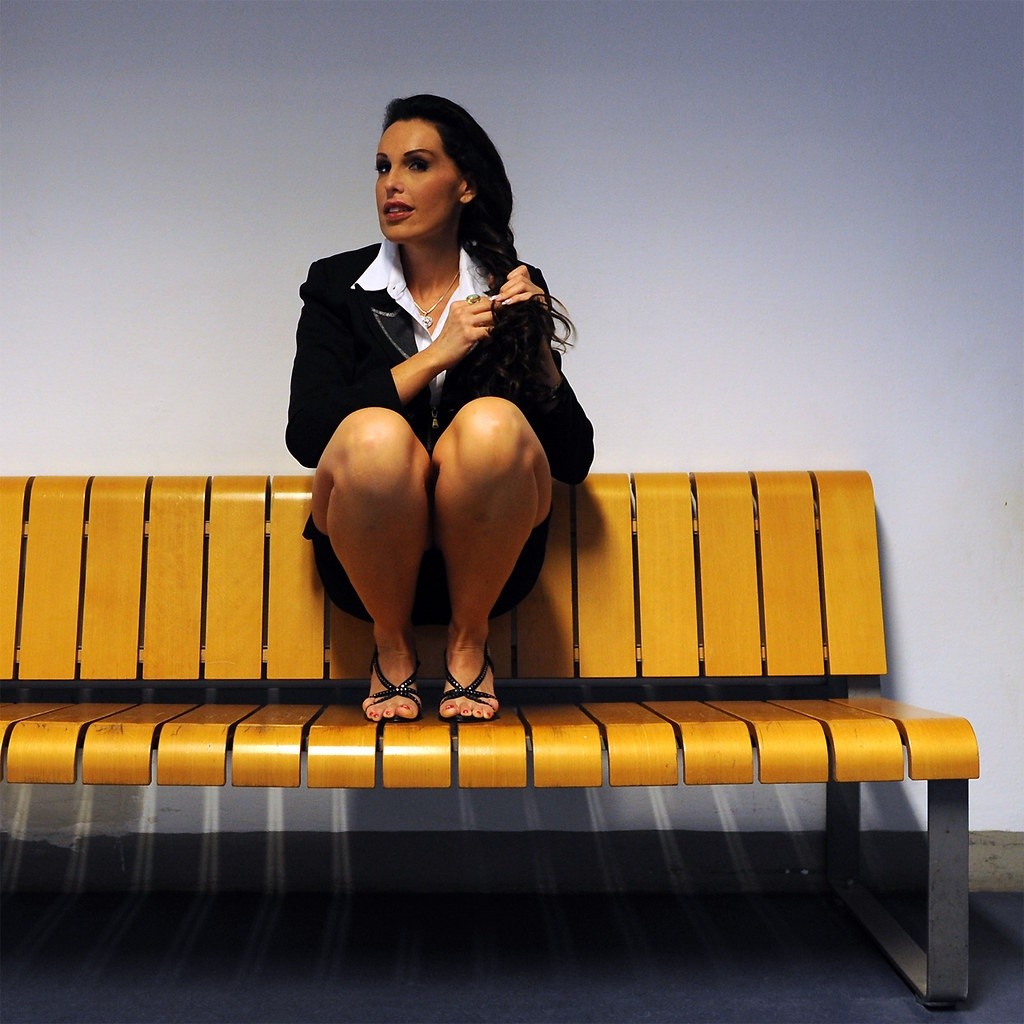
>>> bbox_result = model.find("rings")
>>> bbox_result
[467,293,481,306]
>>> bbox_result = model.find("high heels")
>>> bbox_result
[436,648,500,724]
[358,646,428,726]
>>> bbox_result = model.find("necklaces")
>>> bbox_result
[408,268,462,330]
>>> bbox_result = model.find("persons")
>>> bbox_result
[284,93,597,726]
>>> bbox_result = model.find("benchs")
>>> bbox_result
[0,468,983,1011]
